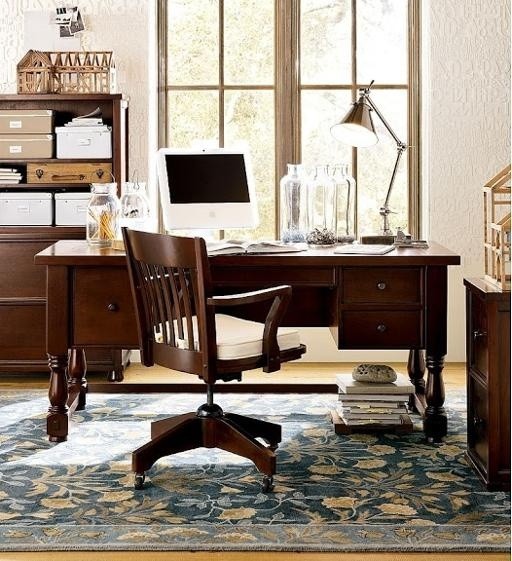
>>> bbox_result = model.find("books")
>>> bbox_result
[66,110,104,127]
[334,242,398,256]
[1,165,24,185]
[205,233,301,259]
[332,375,415,435]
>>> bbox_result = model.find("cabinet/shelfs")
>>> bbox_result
[463,278,511,492]
[0,93,131,381]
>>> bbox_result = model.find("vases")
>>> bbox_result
[280,164,357,242]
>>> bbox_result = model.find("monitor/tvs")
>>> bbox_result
[158,146,262,230]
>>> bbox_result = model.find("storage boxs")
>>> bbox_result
[54,193,93,226]
[55,127,112,160]
[0,110,54,134]
[0,134,53,159]
[26,162,112,184]
[0,193,54,226]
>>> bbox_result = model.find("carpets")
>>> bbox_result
[0,388,511,552]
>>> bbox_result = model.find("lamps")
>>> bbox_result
[330,80,411,236]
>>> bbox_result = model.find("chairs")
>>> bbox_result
[120,227,304,491]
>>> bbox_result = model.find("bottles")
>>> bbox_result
[306,164,339,248]
[86,180,120,248]
[117,179,151,251]
[280,160,309,246]
[331,162,359,241]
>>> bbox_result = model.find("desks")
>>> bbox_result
[33,238,460,441]
